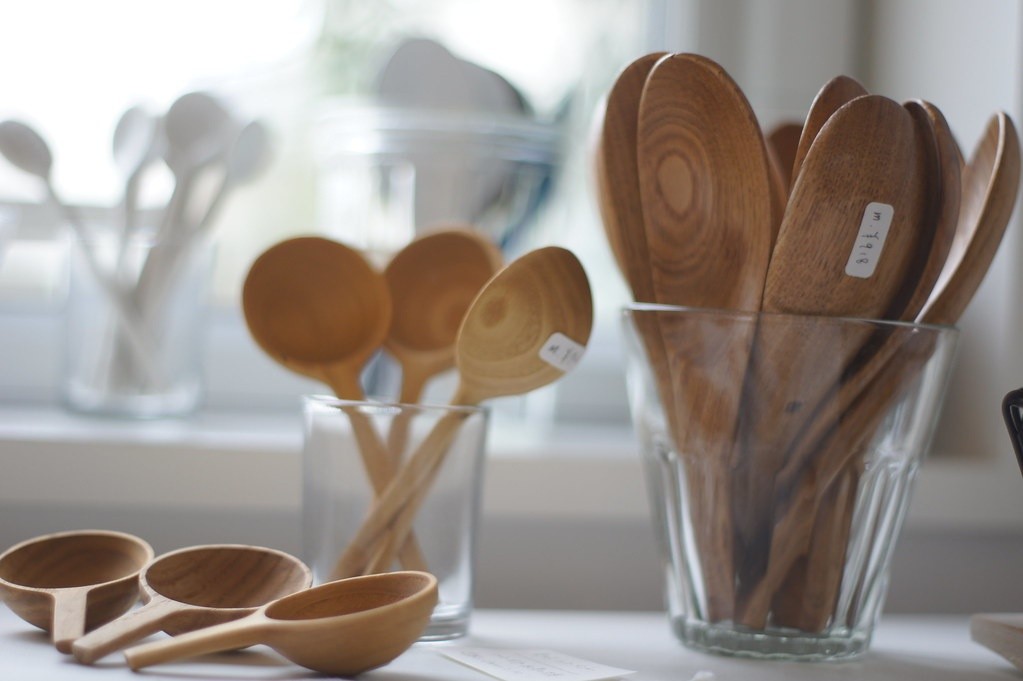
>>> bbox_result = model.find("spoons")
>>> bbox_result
[95,102,166,386]
[73,544,311,666]
[0,531,153,652]
[196,122,270,243]
[242,237,428,571]
[340,246,593,569]
[124,570,437,673]
[371,232,505,500]
[0,121,168,390]
[111,93,235,380]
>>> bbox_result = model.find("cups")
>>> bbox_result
[66,244,211,412]
[620,304,961,658]
[301,394,490,643]
[324,114,555,432]
